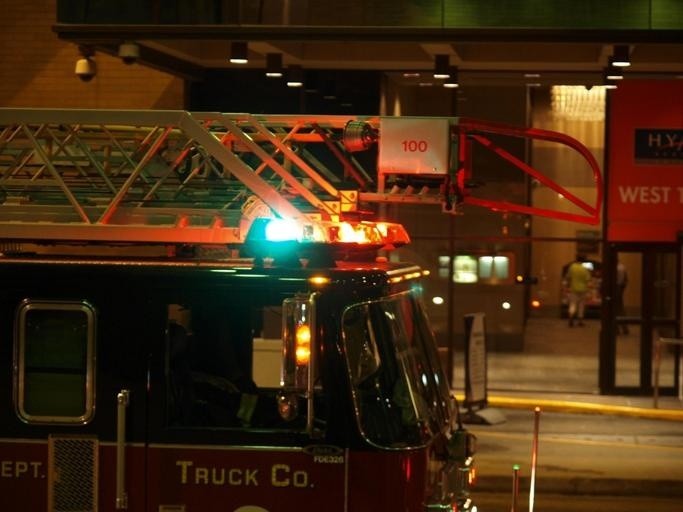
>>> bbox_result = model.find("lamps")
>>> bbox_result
[286,66,354,107]
[229,42,248,65]
[266,53,283,78]
[434,54,451,79]
[443,64,459,88]
[604,45,631,90]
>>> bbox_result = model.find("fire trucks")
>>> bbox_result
[0,99,603,510]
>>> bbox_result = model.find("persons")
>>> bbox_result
[565,253,593,328]
[616,262,630,334]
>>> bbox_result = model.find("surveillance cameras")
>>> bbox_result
[75,58,96,81]
[118,44,140,64]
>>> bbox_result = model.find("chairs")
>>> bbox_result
[164,320,279,425]
